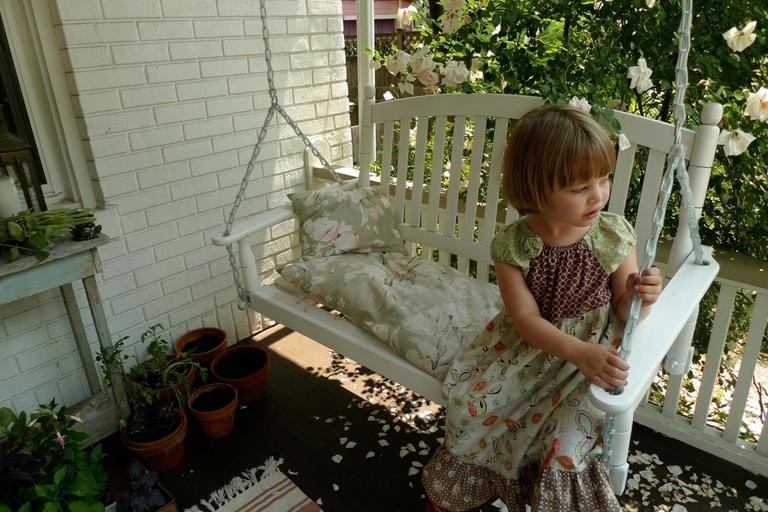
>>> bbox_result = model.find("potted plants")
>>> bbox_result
[94,328,210,473]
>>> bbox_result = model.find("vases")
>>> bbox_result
[175,321,273,440]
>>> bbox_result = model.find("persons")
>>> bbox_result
[421,104,663,511]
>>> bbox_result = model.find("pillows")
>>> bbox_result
[286,183,409,259]
[275,252,503,382]
